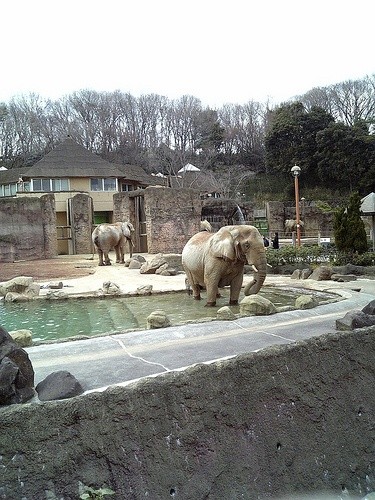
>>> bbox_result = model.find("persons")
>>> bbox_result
[262,234,269,250]
[270,232,279,249]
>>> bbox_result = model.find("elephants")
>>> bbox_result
[181,225,268,306]
[91,222,135,265]
